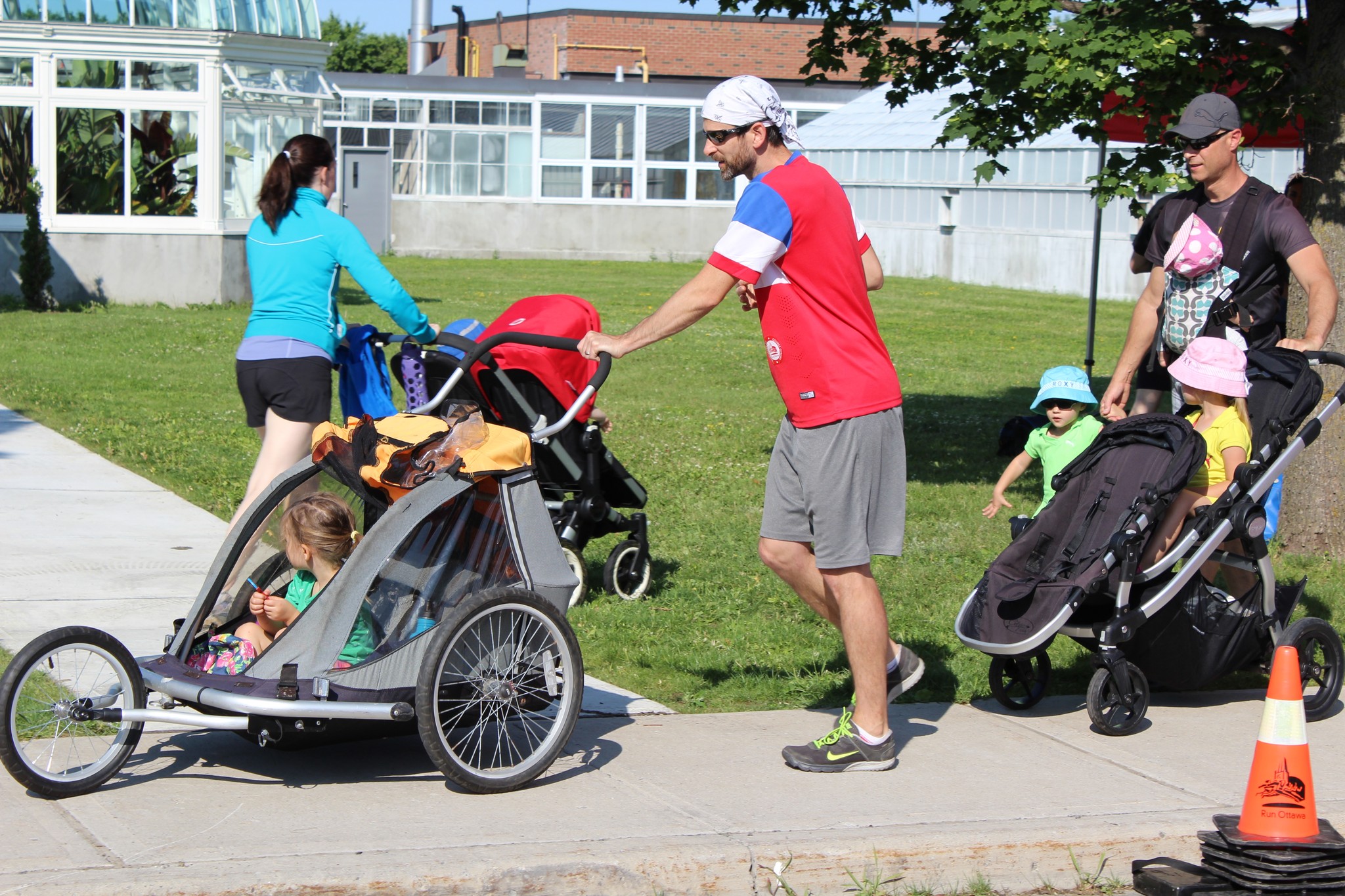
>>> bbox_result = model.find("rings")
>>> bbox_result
[584,351,589,356]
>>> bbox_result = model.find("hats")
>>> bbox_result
[1165,94,1240,140]
[1029,366,1098,416]
[1164,213,1223,279]
[1166,337,1249,397]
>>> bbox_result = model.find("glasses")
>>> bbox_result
[1041,398,1078,411]
[1179,131,1229,150]
[702,117,769,146]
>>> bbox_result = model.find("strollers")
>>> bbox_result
[0,294,655,796]
[955,350,1345,735]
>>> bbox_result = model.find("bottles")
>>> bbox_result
[401,343,430,415]
[409,599,437,639]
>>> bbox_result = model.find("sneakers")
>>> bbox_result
[782,707,896,772]
[834,644,924,730]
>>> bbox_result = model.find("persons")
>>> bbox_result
[577,72,925,772]
[1095,335,1252,596]
[204,133,440,617]
[981,366,1128,522]
[1100,92,1337,601]
[234,491,378,673]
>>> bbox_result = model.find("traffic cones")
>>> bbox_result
[1195,646,1345,896]
